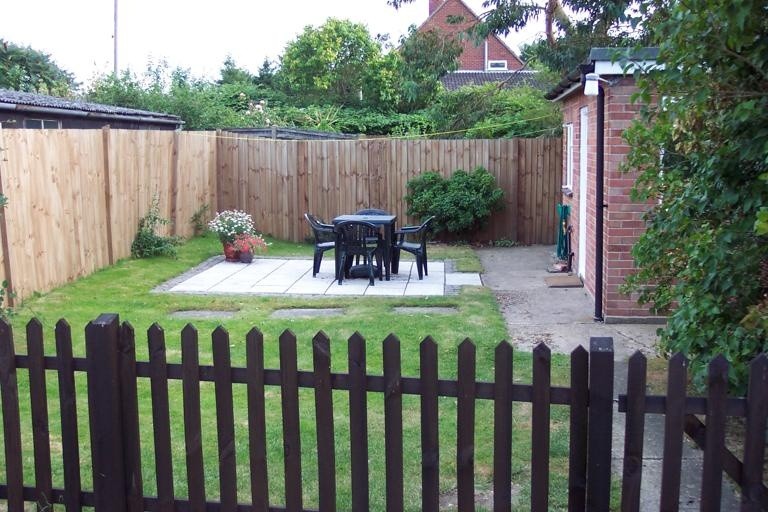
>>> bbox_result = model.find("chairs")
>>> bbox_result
[352,207,398,274]
[332,220,386,286]
[392,214,437,280]
[303,212,345,280]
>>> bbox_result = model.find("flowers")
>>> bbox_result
[231,230,267,256]
[207,207,256,245]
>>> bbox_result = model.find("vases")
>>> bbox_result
[222,233,242,263]
[238,249,253,263]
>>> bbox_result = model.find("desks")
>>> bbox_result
[332,214,398,282]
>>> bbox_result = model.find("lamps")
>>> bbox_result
[581,70,624,98]
